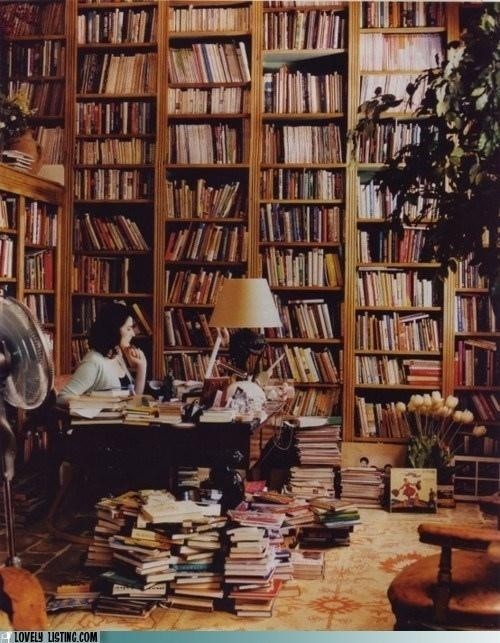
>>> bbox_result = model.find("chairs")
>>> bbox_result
[389,494,498,631]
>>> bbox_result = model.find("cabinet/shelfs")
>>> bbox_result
[1,0,499,502]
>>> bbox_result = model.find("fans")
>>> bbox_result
[0,298,56,615]
